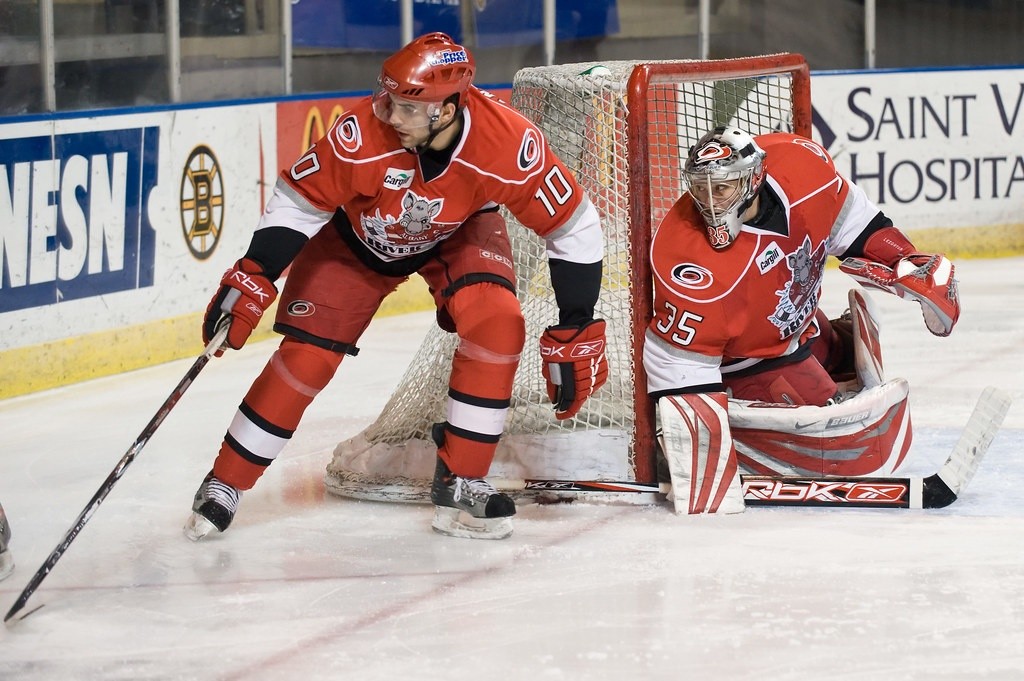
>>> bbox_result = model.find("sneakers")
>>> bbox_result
[182,468,240,541]
[0,503,16,582]
[430,420,517,539]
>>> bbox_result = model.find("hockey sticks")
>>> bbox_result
[3,321,231,626]
[484,386,1013,510]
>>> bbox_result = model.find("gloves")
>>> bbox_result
[202,255,278,357]
[540,318,608,420]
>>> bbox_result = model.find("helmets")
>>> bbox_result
[682,126,767,249]
[380,31,474,110]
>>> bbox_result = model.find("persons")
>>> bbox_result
[643,127,960,514]
[184,33,607,541]
